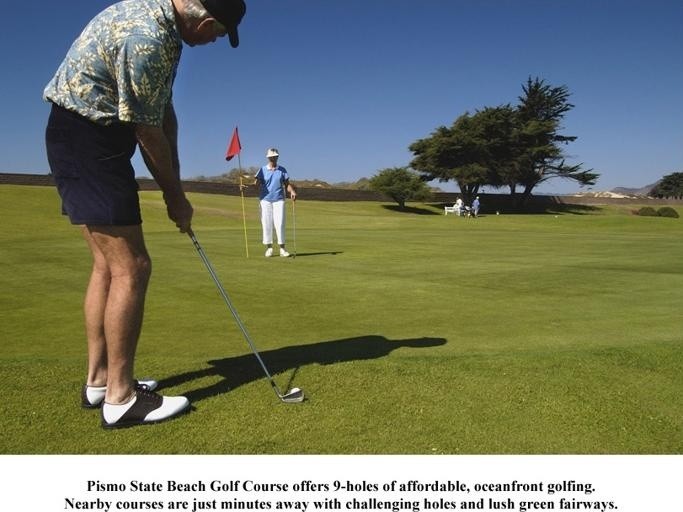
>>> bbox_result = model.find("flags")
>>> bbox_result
[224,127,241,162]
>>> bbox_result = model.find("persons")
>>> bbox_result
[237,147,296,259]
[39,0,248,427]
[455,195,463,215]
[472,196,480,217]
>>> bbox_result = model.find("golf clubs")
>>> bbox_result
[292,194,296,260]
[189,227,305,404]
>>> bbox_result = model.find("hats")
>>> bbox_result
[199,0,246,48]
[266,149,279,158]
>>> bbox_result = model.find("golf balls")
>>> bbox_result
[290,387,300,395]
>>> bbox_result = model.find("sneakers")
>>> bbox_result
[280,248,291,257]
[265,248,274,257]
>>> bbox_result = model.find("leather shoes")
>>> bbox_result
[81,378,158,408]
[101,387,190,429]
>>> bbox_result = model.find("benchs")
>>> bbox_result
[445,207,471,217]
